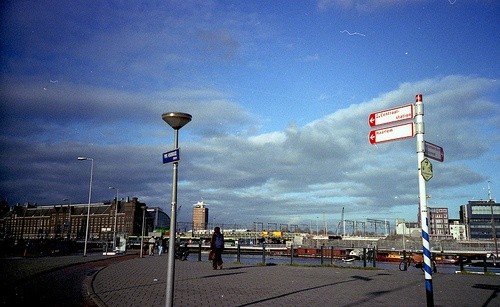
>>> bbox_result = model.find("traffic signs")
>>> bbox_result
[424,140,446,162]
[368,121,414,144]
[368,103,414,128]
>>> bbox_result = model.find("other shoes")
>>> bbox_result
[220,265,223,269]
[213,267,217,270]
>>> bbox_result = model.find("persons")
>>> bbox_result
[175,238,193,261]
[143,236,169,256]
[211,226,225,270]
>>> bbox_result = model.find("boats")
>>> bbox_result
[375,248,461,266]
[342,257,356,262]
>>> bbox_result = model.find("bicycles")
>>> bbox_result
[399,250,437,273]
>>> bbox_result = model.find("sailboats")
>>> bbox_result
[469,181,500,267]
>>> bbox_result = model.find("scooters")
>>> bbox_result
[175,240,189,261]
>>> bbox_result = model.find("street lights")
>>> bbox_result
[140,206,148,258]
[63,198,72,222]
[161,112,193,307]
[77,156,94,257]
[317,217,320,236]
[108,187,119,250]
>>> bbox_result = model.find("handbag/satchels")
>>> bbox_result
[208,251,215,260]
